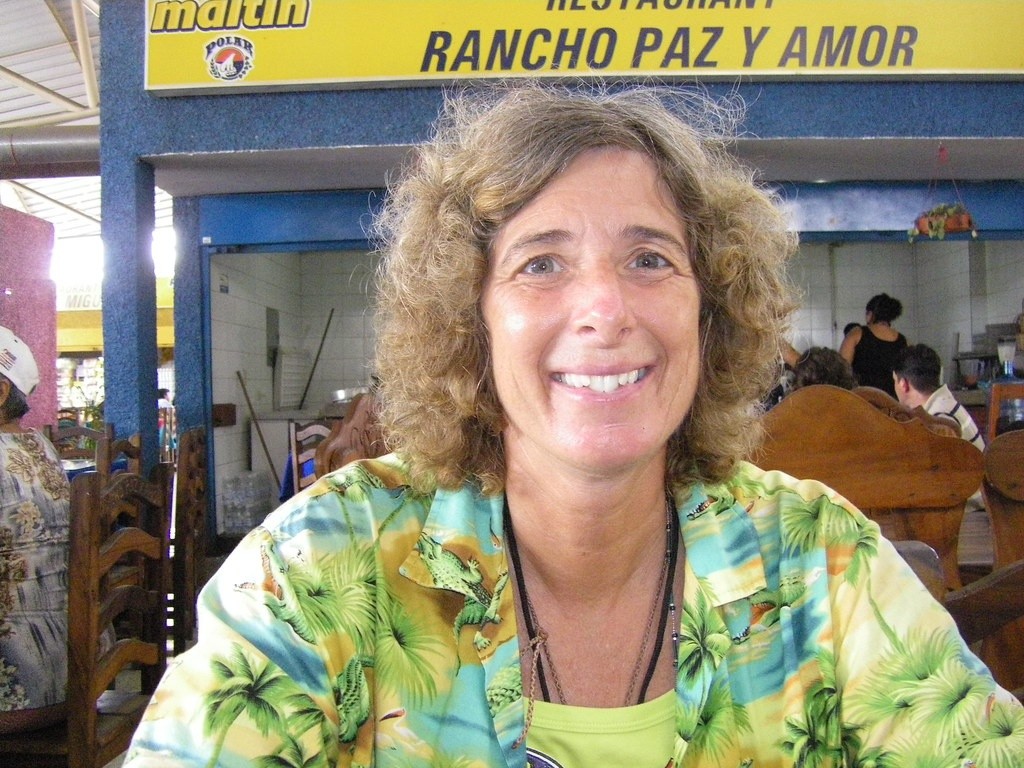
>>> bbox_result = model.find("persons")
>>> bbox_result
[762,336,801,412]
[836,290,908,402]
[0,325,119,731]
[119,86,1024,767]
[158,386,177,471]
[891,342,986,514]
[790,347,854,394]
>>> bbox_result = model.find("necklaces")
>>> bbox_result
[498,486,678,750]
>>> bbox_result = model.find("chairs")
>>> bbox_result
[0,372,1024,767]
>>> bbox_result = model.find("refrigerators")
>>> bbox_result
[250,347,324,482]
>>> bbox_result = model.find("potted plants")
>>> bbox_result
[908,204,979,244]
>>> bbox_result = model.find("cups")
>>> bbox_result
[997,342,1017,368]
[959,360,984,390]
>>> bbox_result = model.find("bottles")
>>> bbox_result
[987,361,1024,440]
[223,472,274,536]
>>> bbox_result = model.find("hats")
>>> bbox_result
[0,325,39,397]
